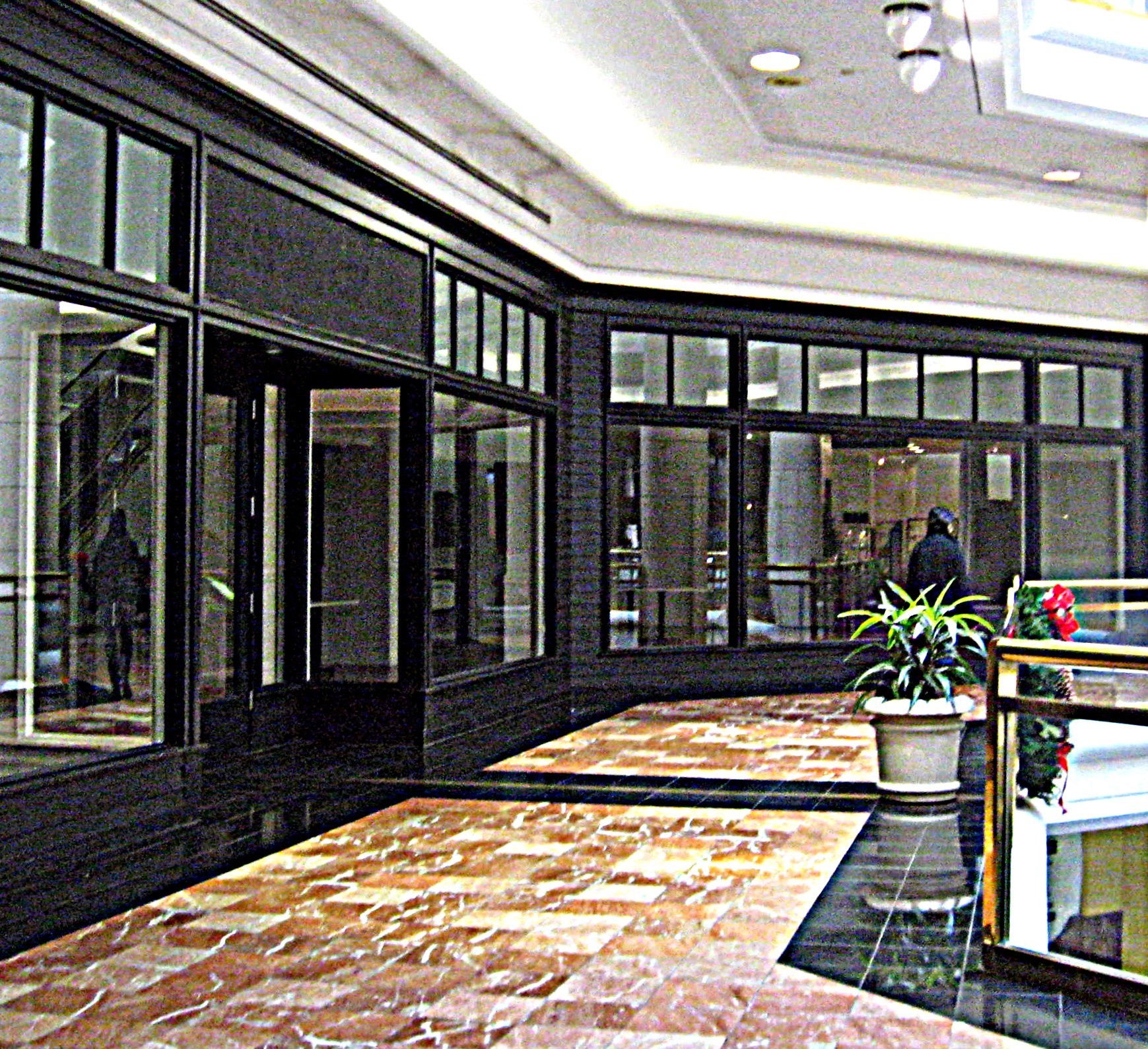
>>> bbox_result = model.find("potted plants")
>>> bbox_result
[849,804,986,996]
[832,571,999,800]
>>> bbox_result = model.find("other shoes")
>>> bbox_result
[108,684,122,701]
[123,681,133,699]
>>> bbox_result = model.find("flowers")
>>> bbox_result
[1011,580,1080,818]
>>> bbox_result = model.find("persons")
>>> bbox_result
[906,506,981,667]
[91,507,150,699]
